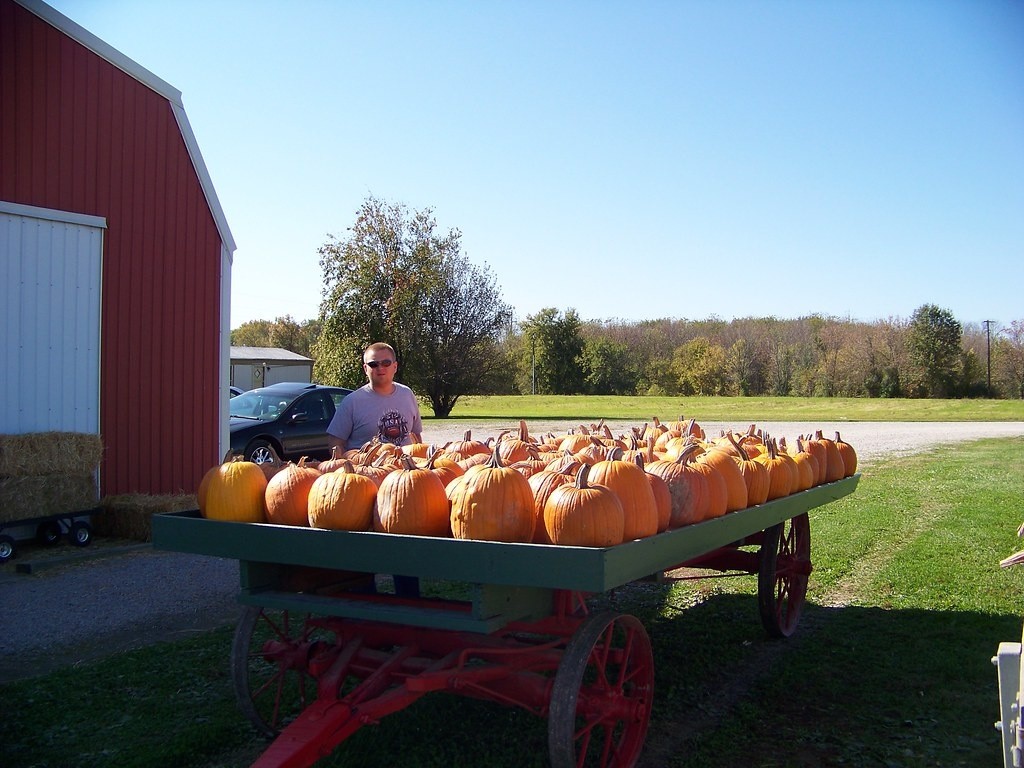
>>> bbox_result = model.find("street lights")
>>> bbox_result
[532,332,537,395]
[984,320,994,400]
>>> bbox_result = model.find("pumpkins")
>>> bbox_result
[198,415,857,545]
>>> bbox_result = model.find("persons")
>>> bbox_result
[327,343,422,597]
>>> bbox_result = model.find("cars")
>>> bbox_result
[229,386,278,417]
[229,382,356,465]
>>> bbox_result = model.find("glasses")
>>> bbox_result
[365,360,396,368]
